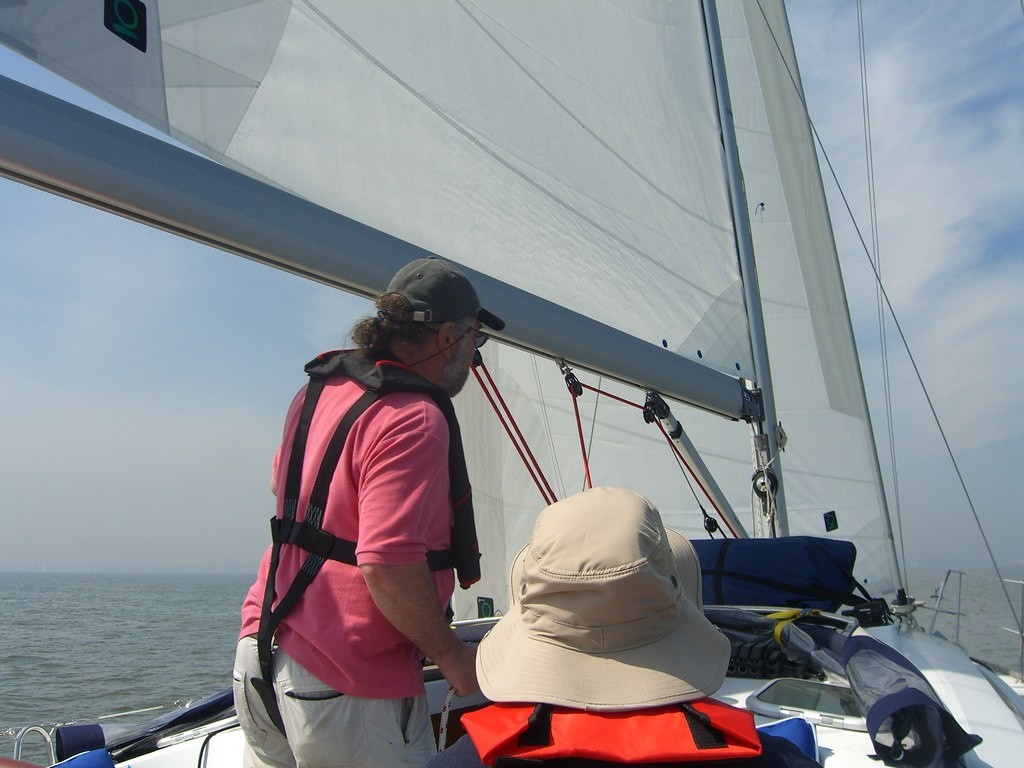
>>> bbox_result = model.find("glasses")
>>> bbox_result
[451,321,490,349]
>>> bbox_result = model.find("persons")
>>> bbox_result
[232,257,506,768]
[426,486,818,768]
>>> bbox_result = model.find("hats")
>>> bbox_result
[476,486,730,712]
[381,255,506,332]
